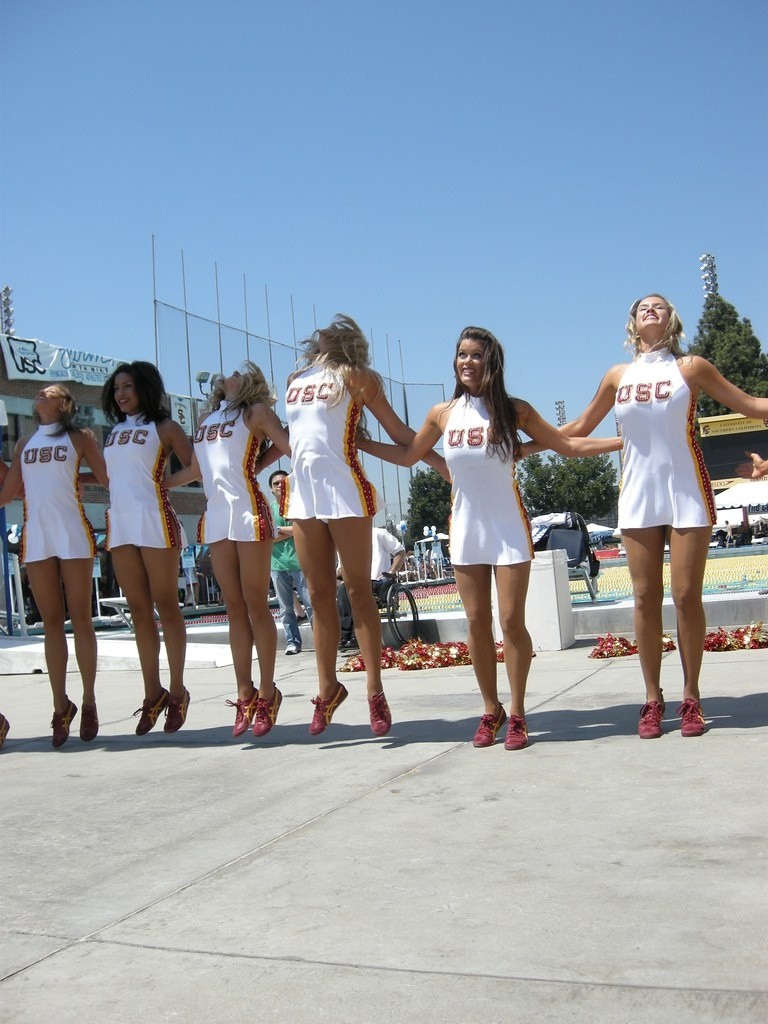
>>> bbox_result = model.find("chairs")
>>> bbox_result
[528,509,603,603]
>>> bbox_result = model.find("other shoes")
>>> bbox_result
[0,713,10,750]
[344,638,358,647]
[337,636,349,647]
[284,644,302,654]
[296,613,309,626]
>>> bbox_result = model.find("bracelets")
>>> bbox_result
[278,526,281,531]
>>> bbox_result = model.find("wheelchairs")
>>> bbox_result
[336,571,420,652]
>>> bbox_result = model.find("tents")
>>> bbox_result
[585,522,615,532]
[415,533,450,581]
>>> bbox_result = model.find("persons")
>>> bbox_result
[161,358,291,737]
[270,471,313,655]
[738,521,746,544]
[516,293,768,739]
[75,359,202,736]
[0,383,110,747]
[354,326,624,751]
[725,520,732,548]
[758,522,768,534]
[336,524,406,651]
[255,314,452,737]
[734,451,768,479]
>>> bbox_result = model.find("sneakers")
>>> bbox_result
[308,681,348,736]
[131,686,169,736]
[472,701,506,747]
[505,715,528,750]
[251,682,283,737]
[164,685,190,734]
[368,690,391,735]
[51,694,78,748]
[225,682,259,737]
[80,702,98,741]
[639,688,665,739]
[676,696,705,736]
